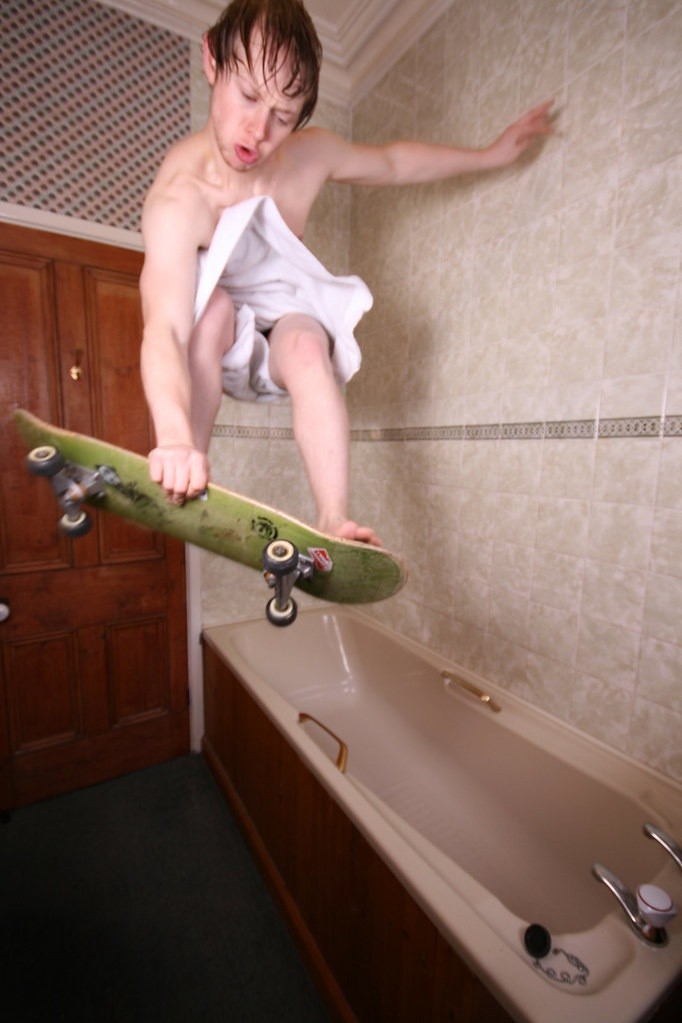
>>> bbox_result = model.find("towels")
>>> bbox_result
[195,194,376,406]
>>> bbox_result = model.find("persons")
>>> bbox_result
[137,0,554,553]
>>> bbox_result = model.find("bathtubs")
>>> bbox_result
[200,600,682,1023]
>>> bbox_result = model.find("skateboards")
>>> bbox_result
[9,405,409,629]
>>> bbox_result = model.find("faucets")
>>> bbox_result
[588,860,677,951]
[641,822,682,871]
